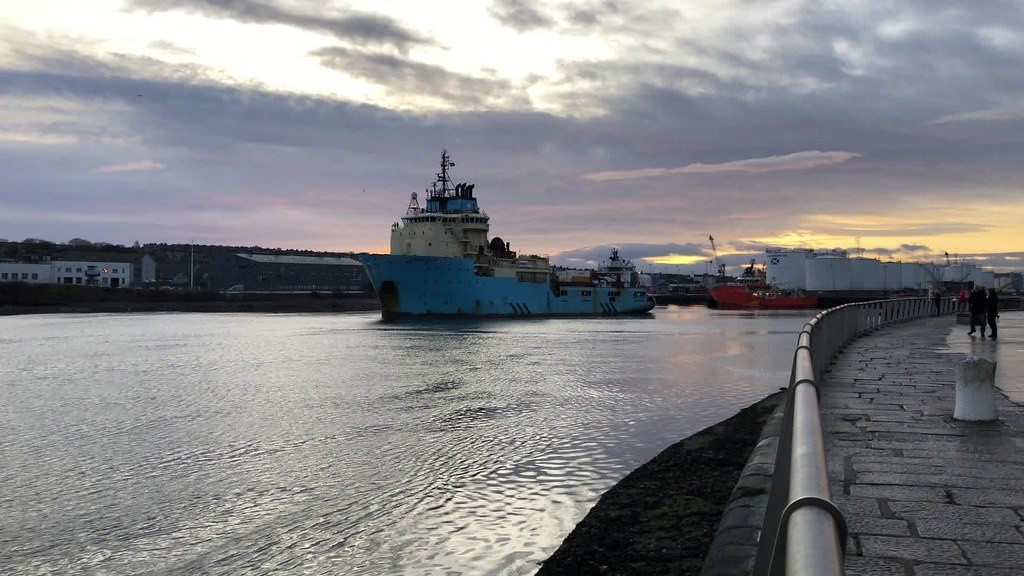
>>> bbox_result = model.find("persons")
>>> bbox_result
[960,284,999,340]
[932,289,941,317]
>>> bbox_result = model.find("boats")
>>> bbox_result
[702,257,820,311]
[356,146,657,323]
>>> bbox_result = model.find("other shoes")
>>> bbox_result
[982,333,985,337]
[968,330,976,334]
[988,335,997,340]
